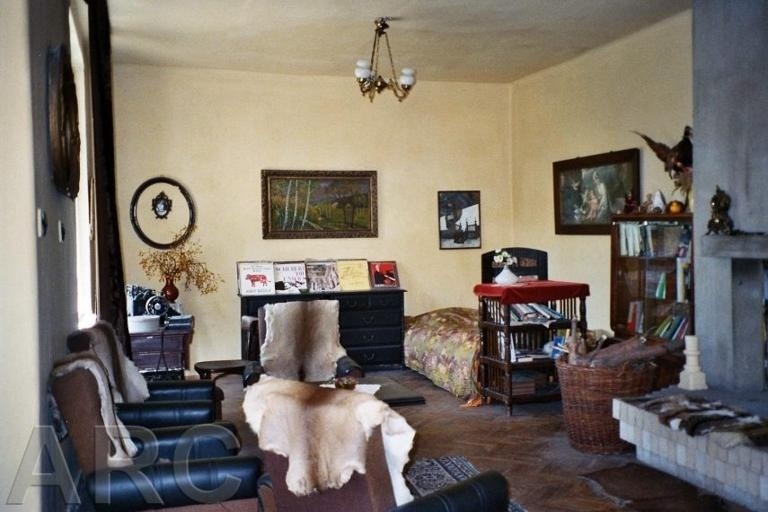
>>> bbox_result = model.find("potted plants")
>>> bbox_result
[138,223,227,303]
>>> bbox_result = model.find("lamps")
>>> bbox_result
[354,16,417,102]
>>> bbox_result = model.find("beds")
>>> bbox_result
[403,306,598,399]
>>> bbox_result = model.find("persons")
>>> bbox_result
[586,169,610,220]
[374,264,387,284]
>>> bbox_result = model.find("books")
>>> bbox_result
[487,299,570,396]
[627,300,691,342]
[619,220,691,258]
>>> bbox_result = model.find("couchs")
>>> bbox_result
[235,376,510,511]
[49,356,278,512]
[244,300,362,389]
[66,321,224,428]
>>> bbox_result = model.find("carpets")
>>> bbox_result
[402,454,528,511]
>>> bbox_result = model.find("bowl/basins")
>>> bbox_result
[335,377,358,389]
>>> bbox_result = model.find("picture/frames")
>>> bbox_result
[552,148,643,235]
[438,190,482,249]
[260,168,379,238]
[369,261,400,289]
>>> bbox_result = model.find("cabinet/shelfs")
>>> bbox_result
[610,214,695,342]
[473,279,590,415]
[237,287,407,368]
[131,315,193,370]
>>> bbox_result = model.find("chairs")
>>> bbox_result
[194,315,257,390]
